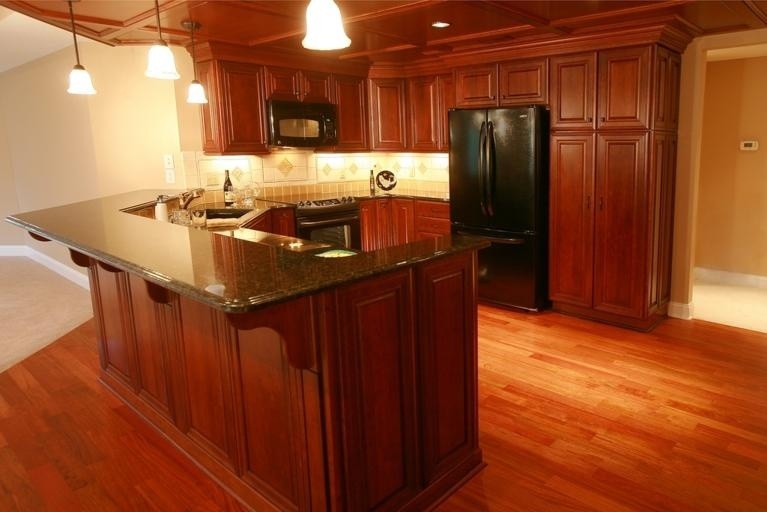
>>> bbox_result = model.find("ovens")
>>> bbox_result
[296,212,360,251]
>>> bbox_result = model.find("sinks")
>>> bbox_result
[206,208,252,218]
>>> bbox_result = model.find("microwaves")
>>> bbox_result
[266,99,338,148]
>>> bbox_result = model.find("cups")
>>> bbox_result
[190,208,208,230]
[170,209,189,226]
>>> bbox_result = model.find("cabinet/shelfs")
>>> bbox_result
[316,74,365,153]
[412,201,451,242]
[360,200,414,251]
[548,46,680,129]
[196,58,265,155]
[244,210,297,237]
[451,55,547,109]
[368,72,451,153]
[265,64,327,103]
[549,133,677,333]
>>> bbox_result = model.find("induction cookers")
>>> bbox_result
[253,192,357,205]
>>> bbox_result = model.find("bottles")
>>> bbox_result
[369,169,376,197]
[223,169,235,209]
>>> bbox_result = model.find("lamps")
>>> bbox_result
[142,0,179,80]
[182,21,210,104]
[298,0,352,53]
[60,0,96,96]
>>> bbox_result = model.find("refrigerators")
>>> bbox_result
[445,105,552,315]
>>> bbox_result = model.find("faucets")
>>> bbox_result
[178,187,205,210]
